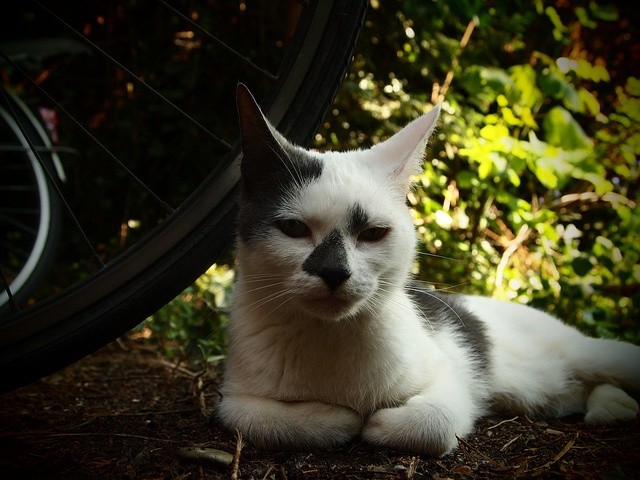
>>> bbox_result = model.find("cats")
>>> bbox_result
[215,82,640,460]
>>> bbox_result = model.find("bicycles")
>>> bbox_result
[0,0,367,388]
